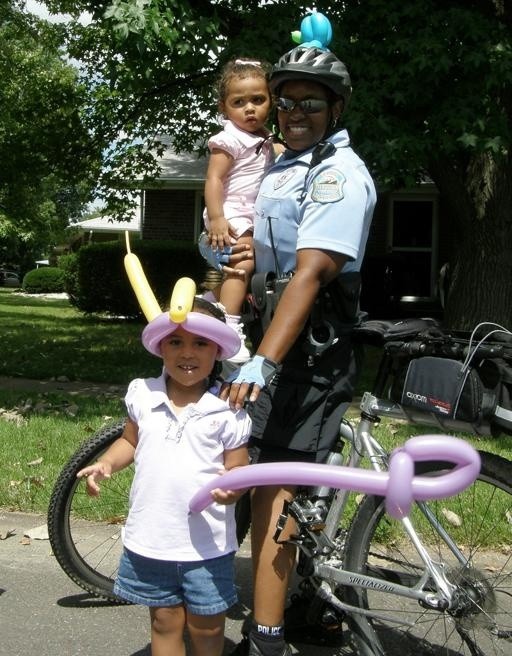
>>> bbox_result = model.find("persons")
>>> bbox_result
[75,297,253,656]
[218,46,378,655]
[201,57,287,366]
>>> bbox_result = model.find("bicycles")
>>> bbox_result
[46,295,512,656]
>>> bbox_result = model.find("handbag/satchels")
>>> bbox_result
[402,356,501,426]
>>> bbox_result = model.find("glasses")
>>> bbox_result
[275,95,331,114]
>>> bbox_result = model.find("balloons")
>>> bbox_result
[123,230,242,362]
[291,13,334,50]
[187,432,483,519]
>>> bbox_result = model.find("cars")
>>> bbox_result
[0,271,20,287]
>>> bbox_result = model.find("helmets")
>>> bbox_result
[268,45,352,104]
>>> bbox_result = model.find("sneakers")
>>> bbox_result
[231,636,293,656]
[222,322,250,363]
